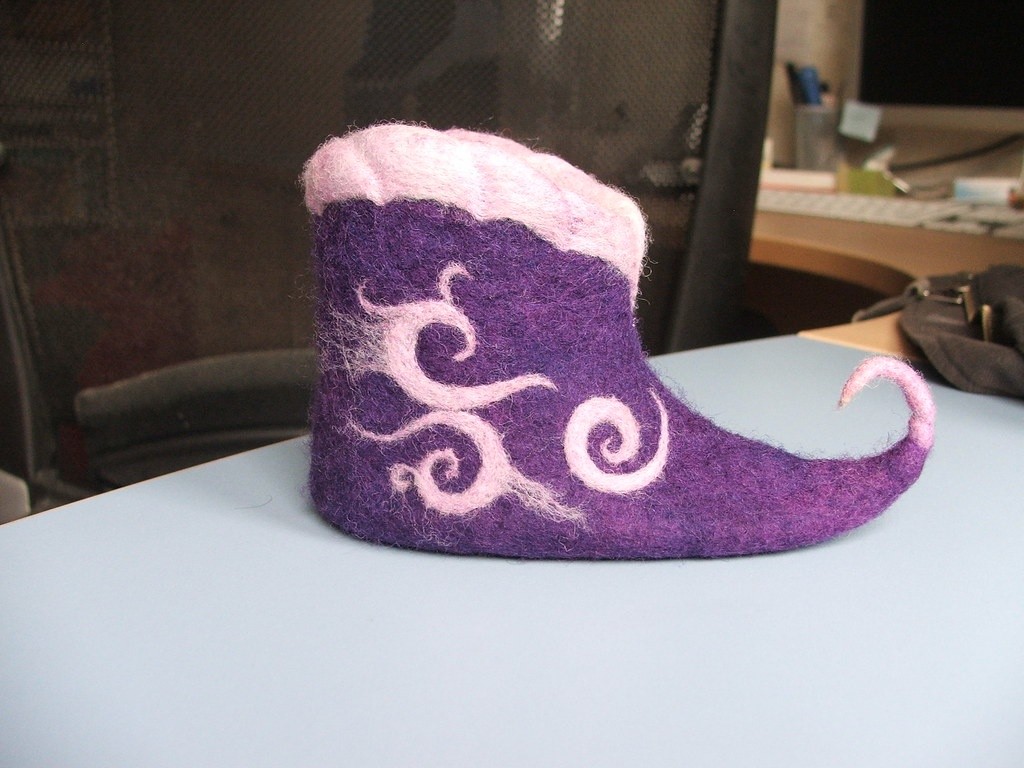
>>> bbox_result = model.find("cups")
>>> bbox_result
[837,164,895,197]
[794,105,848,172]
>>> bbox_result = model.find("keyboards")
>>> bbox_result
[755,189,972,228]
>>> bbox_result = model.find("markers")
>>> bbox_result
[799,68,821,104]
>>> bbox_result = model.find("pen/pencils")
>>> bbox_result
[785,62,807,104]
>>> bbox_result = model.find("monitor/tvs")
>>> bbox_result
[846,0,1024,137]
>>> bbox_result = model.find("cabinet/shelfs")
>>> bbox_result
[1,0,124,227]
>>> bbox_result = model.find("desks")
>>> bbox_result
[0,127,1022,766]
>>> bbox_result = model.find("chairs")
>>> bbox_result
[1,0,777,519]
